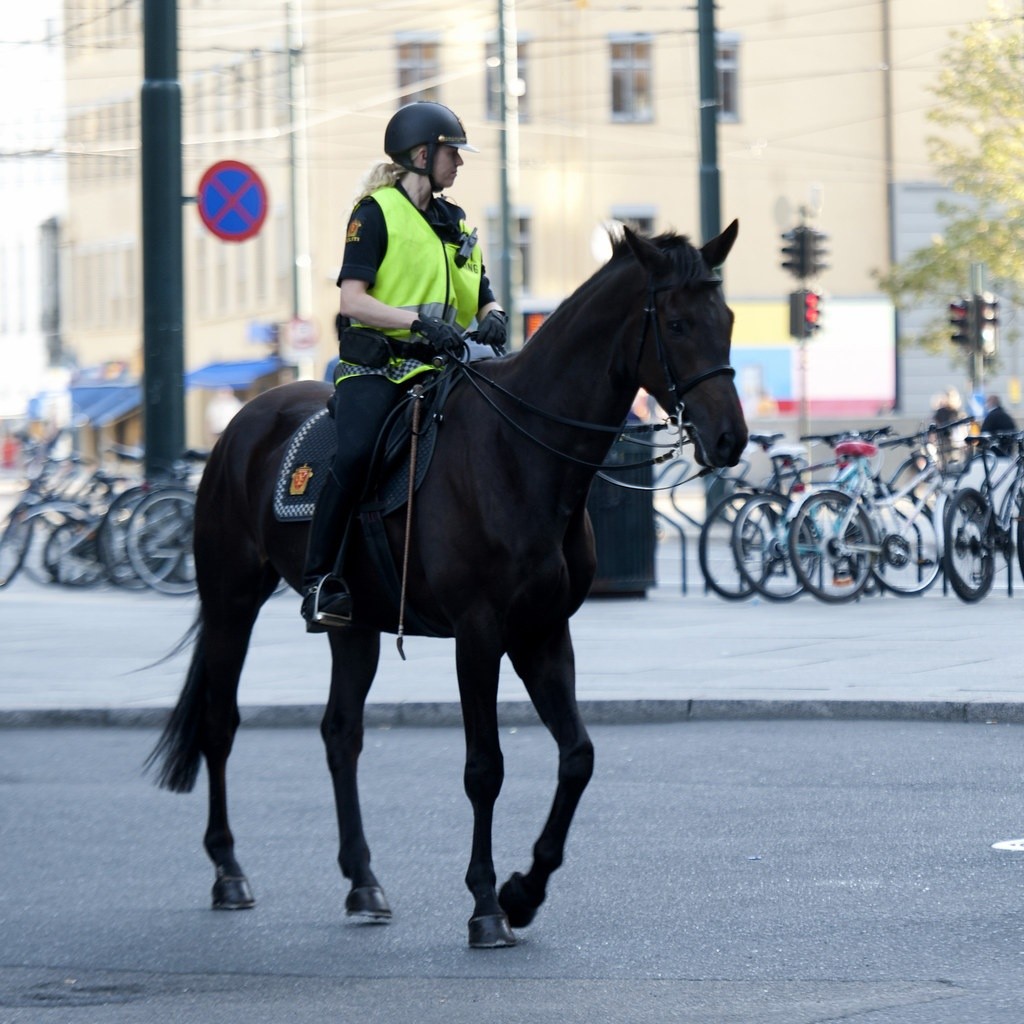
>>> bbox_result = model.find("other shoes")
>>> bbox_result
[300,575,356,635]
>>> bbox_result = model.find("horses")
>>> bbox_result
[122,218,749,948]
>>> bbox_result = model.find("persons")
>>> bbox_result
[207,386,242,440]
[915,394,1015,505]
[296,101,509,635]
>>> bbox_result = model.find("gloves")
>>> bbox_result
[411,312,467,358]
[471,309,509,347]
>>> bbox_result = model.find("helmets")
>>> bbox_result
[384,100,482,157]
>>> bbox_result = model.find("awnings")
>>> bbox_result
[182,356,296,388]
[31,381,142,427]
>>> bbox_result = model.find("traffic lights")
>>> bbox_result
[949,300,975,348]
[976,296,999,333]
[797,290,821,336]
[780,231,803,276]
[804,229,828,274]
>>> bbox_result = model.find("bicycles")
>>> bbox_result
[787,416,987,601]
[699,432,876,600]
[943,432,1024,603]
[732,427,940,596]
[1,432,290,596]
